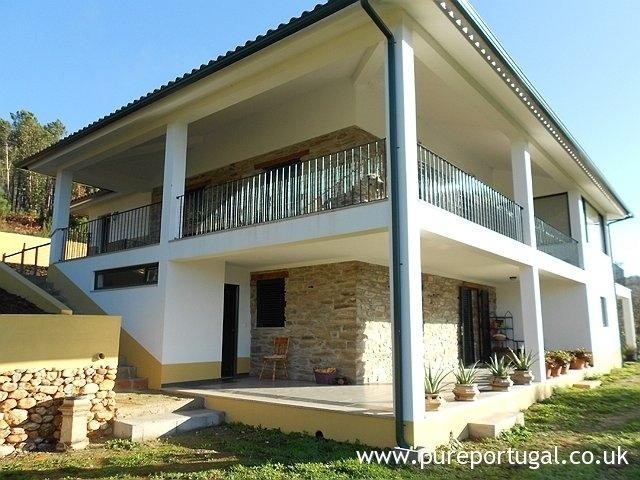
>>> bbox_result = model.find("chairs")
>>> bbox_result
[258,336,291,382]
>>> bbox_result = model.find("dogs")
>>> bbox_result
[324,173,384,210]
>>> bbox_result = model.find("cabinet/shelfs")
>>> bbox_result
[489,310,515,367]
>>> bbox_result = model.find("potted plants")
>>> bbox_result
[545,344,592,378]
[622,344,639,362]
[425,345,539,413]
